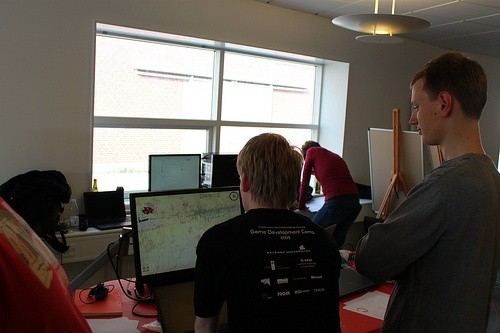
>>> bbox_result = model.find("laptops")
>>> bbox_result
[83,191,132,230]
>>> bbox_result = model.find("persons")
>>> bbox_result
[354,52,500,333]
[193,133,341,333]
[298,141,362,247]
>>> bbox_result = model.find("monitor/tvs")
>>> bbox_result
[129,186,245,283]
[149,154,201,191]
[211,155,240,188]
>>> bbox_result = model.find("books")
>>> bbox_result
[344,289,391,320]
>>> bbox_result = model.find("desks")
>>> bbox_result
[74,252,395,333]
[41,195,372,264]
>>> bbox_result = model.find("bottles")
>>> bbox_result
[92,179,98,192]
[314,178,321,194]
[69,198,80,231]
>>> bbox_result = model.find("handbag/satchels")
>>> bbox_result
[0,169,72,254]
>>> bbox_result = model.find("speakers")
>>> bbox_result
[79,214,88,232]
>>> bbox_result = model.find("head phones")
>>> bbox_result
[87,284,109,301]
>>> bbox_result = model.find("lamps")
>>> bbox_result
[332,0,432,45]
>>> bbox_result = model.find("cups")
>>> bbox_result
[339,250,350,261]
[79,214,88,232]
